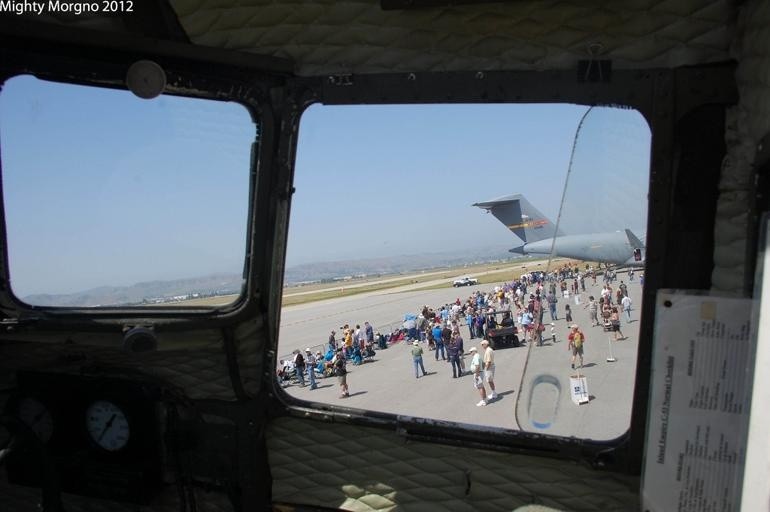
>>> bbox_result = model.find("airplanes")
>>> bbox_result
[470,193,647,267]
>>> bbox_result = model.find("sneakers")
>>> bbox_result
[476,391,499,407]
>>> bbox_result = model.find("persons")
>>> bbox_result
[387,262,645,378]
[480,340,498,400]
[334,353,349,399]
[277,322,386,391]
[469,347,488,406]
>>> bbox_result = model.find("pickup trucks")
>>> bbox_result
[453,276,477,286]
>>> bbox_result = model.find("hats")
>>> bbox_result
[280,292,618,365]
